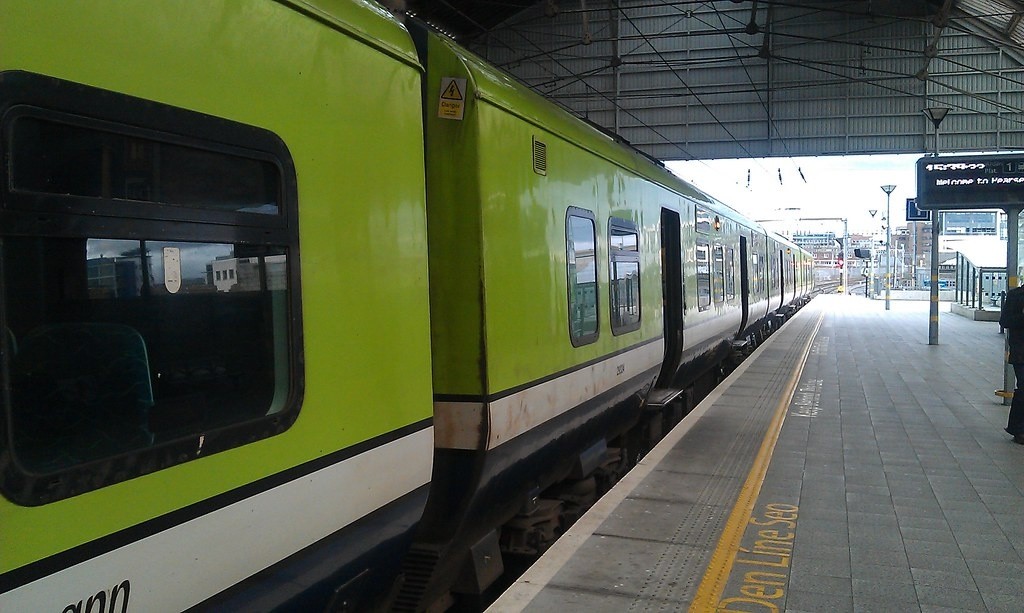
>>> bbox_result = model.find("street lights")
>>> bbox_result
[921,106,953,345]
[880,185,898,310]
[868,210,878,299]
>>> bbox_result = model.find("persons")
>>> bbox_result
[998,284,1024,444]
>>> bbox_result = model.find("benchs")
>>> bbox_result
[31,320,160,423]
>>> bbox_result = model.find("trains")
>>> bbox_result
[0,0,817,613]
